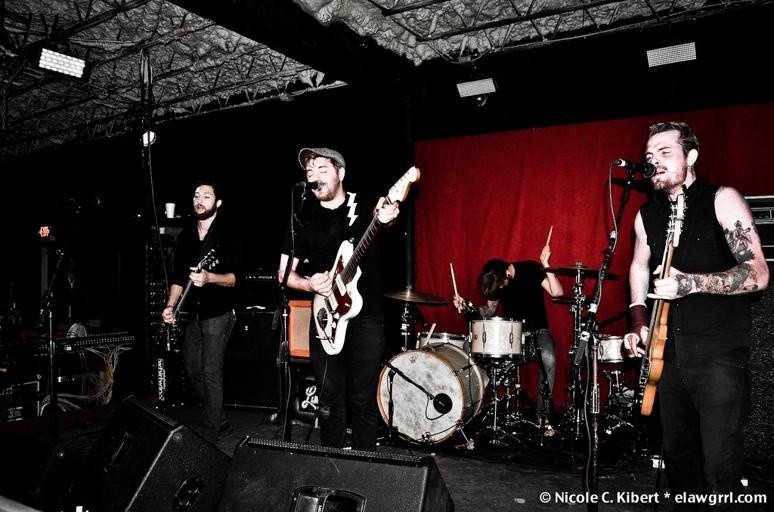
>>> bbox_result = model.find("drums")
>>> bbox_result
[590,335,628,364]
[469,317,523,357]
[417,332,468,356]
[376,342,483,447]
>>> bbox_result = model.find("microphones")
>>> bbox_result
[615,160,656,180]
[297,179,325,187]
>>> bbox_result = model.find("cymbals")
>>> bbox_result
[383,287,448,307]
[538,262,619,280]
[552,293,591,305]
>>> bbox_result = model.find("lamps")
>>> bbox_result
[23,40,94,83]
[133,104,161,147]
[455,77,499,108]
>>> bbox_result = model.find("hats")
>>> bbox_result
[298,148,346,171]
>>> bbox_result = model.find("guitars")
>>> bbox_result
[156,247,219,353]
[311,166,421,357]
[634,194,688,416]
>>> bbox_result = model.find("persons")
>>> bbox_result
[159,179,239,436]
[279,146,401,452]
[452,244,565,440]
[624,123,770,503]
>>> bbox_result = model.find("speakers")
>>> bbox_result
[221,435,456,511]
[91,394,228,510]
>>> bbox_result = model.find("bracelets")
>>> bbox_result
[165,305,177,308]
